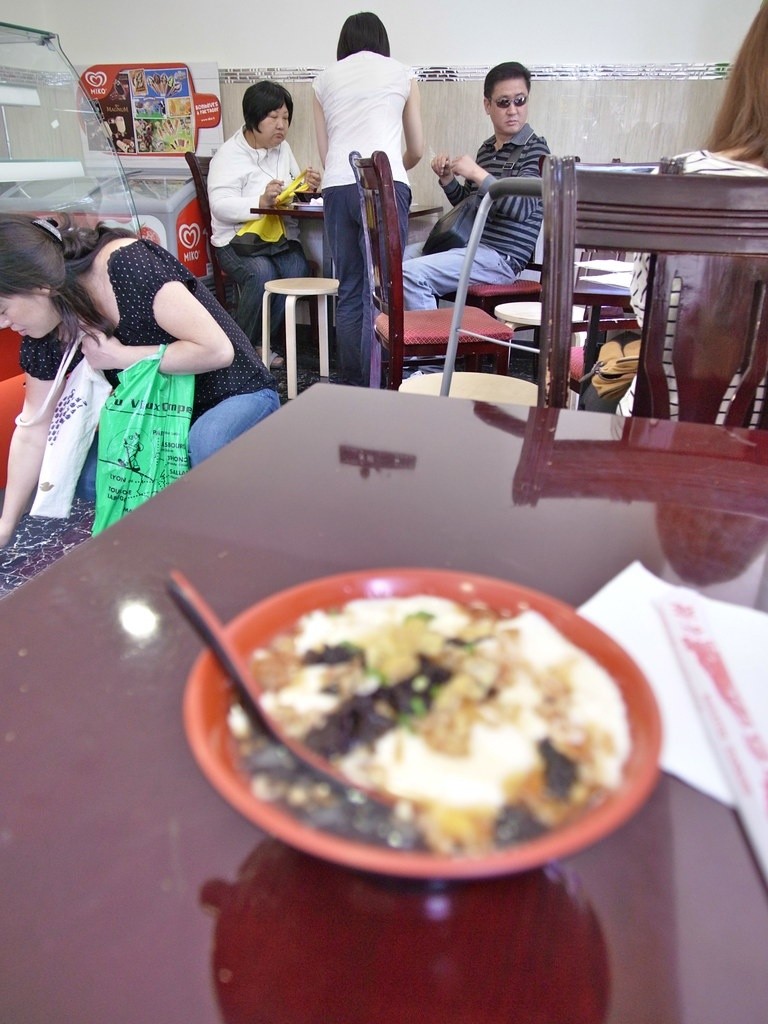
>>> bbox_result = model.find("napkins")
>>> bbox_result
[577,560,767,806]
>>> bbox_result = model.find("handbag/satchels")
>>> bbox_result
[230,169,310,256]
[15,331,113,521]
[90,344,194,537]
[422,196,480,254]
[577,332,641,413]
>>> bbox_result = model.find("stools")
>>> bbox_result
[398,370,540,407]
[494,301,587,349]
[262,277,341,400]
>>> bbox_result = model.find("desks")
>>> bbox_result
[1,380,768,1024]
[250,205,444,364]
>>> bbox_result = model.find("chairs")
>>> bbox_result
[543,151,768,429]
[347,149,512,394]
[187,150,320,357]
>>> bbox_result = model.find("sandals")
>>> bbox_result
[255,346,284,368]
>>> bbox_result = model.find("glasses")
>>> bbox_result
[495,95,526,108]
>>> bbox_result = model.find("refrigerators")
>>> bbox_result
[0,168,237,322]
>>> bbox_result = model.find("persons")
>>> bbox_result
[313,11,423,392]
[0,213,279,548]
[401,63,553,378]
[207,83,321,368]
[577,0,768,433]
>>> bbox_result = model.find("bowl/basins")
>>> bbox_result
[186,569,662,877]
[294,192,322,202]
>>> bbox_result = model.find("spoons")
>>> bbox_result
[163,568,396,805]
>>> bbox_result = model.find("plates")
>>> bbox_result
[290,202,324,212]
